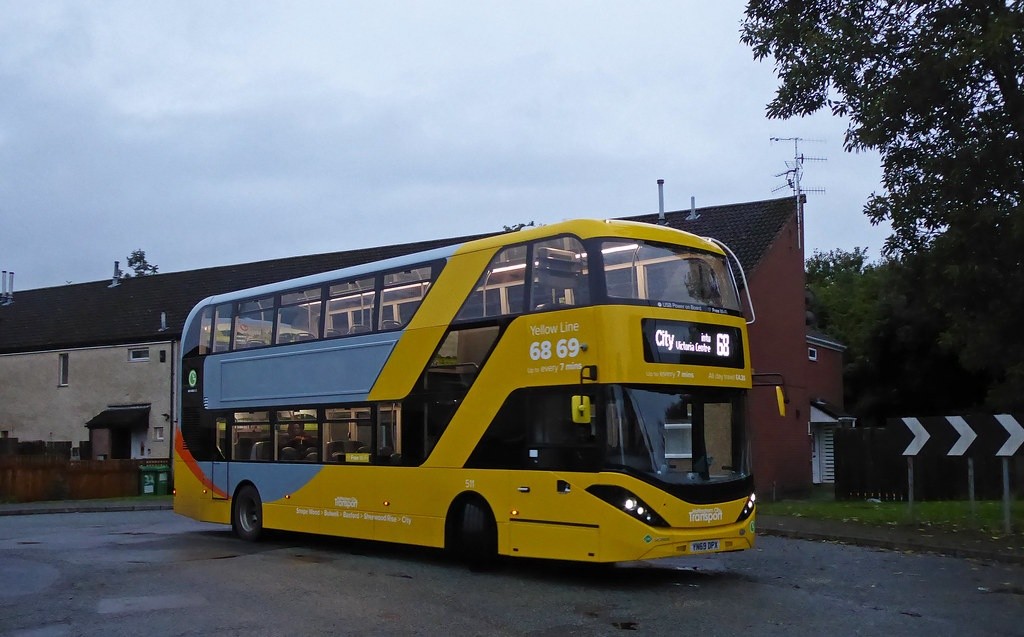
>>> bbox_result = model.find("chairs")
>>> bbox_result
[248,441,271,464]
[327,327,342,338]
[246,338,265,348]
[306,439,402,467]
[380,321,402,331]
[280,333,316,343]
[279,448,301,465]
[347,325,373,334]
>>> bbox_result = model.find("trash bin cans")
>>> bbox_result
[155,466,171,495]
[137,466,158,497]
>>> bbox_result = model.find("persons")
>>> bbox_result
[284,421,312,444]
[574,410,665,472]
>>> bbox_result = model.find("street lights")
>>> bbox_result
[158,311,175,496]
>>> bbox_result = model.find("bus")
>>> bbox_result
[169,217,790,564]
